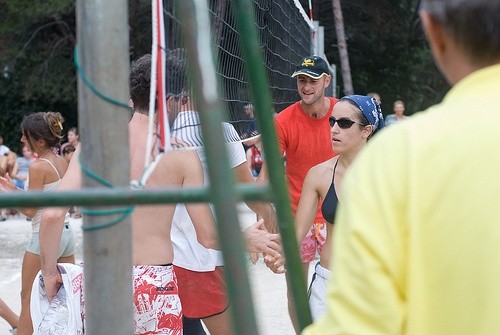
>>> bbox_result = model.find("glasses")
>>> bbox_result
[407,0,432,50]
[329,116,364,130]
[172,94,188,105]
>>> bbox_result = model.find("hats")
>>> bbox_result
[291,56,331,79]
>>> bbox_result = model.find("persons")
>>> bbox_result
[300,0,500,335]
[60,128,81,154]
[265,95,386,323]
[12,145,37,189]
[63,145,75,161]
[0,136,17,176]
[241,103,259,138]
[0,112,75,335]
[385,100,406,125]
[170,48,281,335]
[256,56,339,335]
[39,53,283,335]
